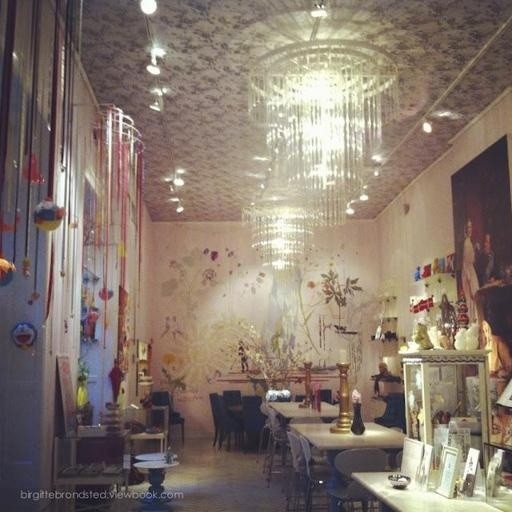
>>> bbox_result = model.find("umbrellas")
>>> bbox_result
[107,358,125,403]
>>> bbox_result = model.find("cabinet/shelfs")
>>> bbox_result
[75,167,108,385]
[52,428,131,510]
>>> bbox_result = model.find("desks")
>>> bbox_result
[353,470,512,512]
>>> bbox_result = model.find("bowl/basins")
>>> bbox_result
[388,473,411,490]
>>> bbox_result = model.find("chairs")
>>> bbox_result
[208,389,404,511]
[151,390,185,443]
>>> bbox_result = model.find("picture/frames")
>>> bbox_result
[401,432,481,498]
[57,353,78,434]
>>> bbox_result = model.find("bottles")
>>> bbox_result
[412,254,445,281]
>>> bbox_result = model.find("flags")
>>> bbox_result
[0,198,129,351]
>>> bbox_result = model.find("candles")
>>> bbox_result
[339,350,347,364]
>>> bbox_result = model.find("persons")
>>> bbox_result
[459,215,512,378]
